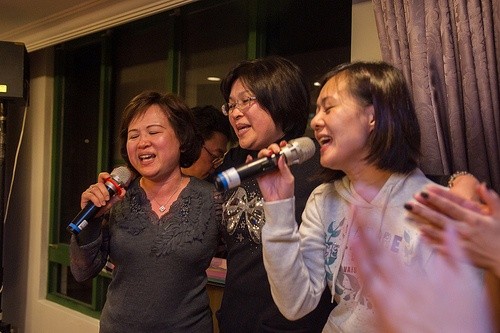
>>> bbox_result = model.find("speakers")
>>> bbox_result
[0,41,29,108]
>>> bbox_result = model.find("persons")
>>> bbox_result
[69,57,500,333]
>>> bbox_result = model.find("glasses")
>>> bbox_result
[220,93,257,116]
[202,145,223,169]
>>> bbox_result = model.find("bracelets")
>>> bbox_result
[447,170,468,188]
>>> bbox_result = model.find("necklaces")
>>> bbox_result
[143,181,181,213]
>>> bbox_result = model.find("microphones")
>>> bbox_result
[214,137,316,193]
[65,166,130,237]
